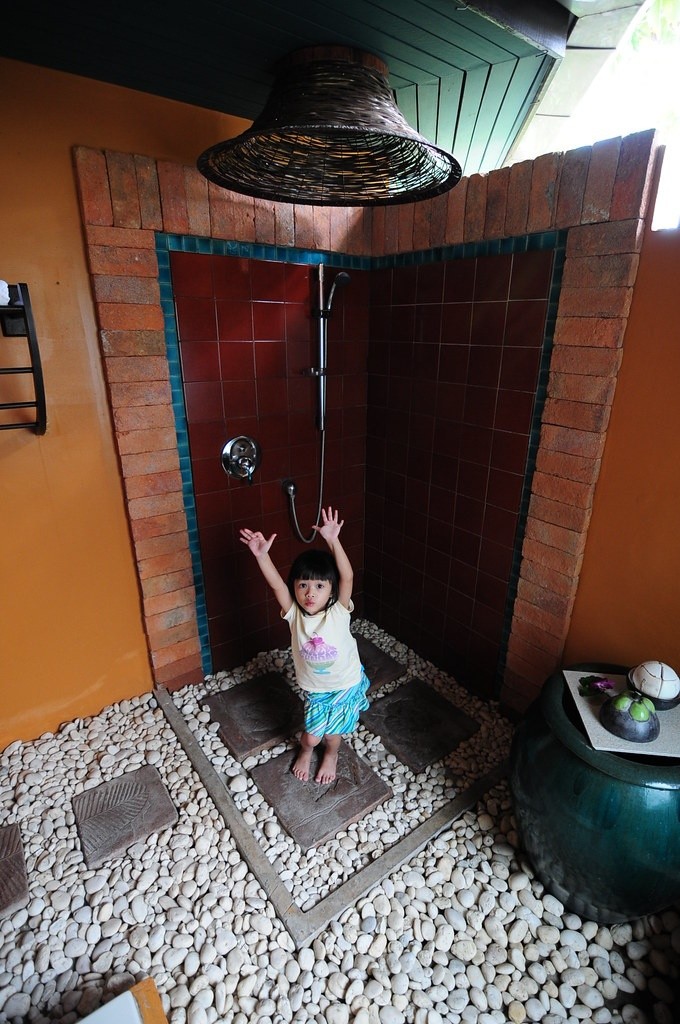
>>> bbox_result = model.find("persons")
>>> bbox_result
[239,505,370,784]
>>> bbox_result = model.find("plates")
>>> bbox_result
[563,669,680,759]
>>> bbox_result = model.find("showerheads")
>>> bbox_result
[327,272,349,312]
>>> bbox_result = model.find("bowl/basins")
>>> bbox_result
[598,692,661,743]
[626,660,680,711]
